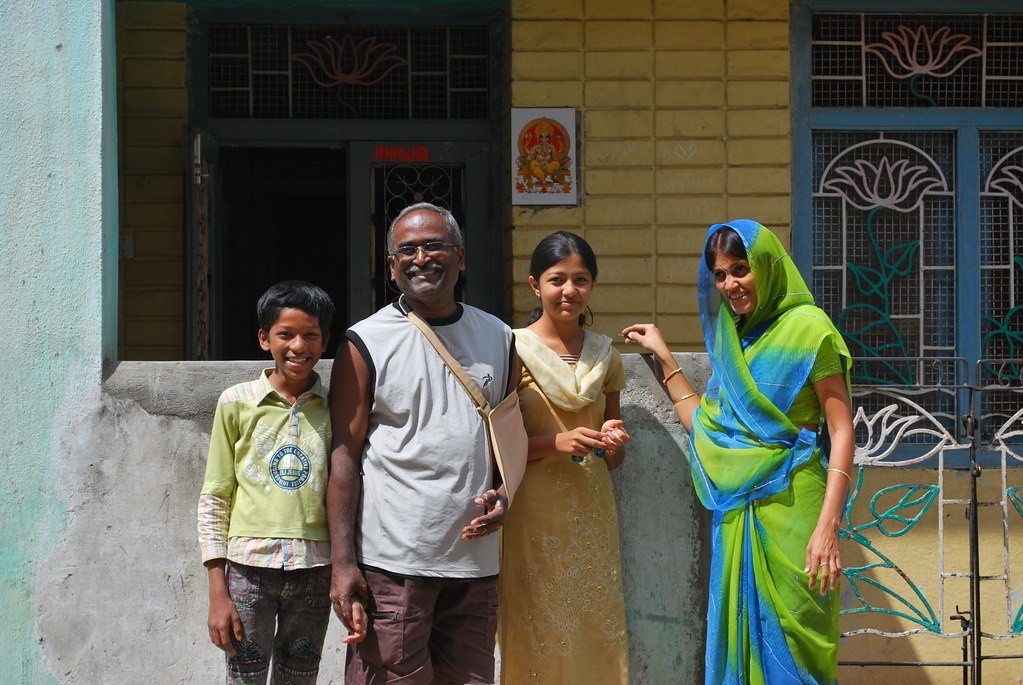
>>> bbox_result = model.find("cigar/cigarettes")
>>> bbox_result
[617,333,629,338]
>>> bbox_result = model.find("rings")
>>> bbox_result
[820,563,829,567]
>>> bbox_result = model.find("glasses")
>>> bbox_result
[390,241,461,258]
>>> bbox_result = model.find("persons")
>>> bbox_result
[499,231,631,684]
[196,282,369,685]
[329,203,518,685]
[622,218,855,685]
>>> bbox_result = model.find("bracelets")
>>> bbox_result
[663,368,683,384]
[673,392,697,404]
[828,469,852,484]
